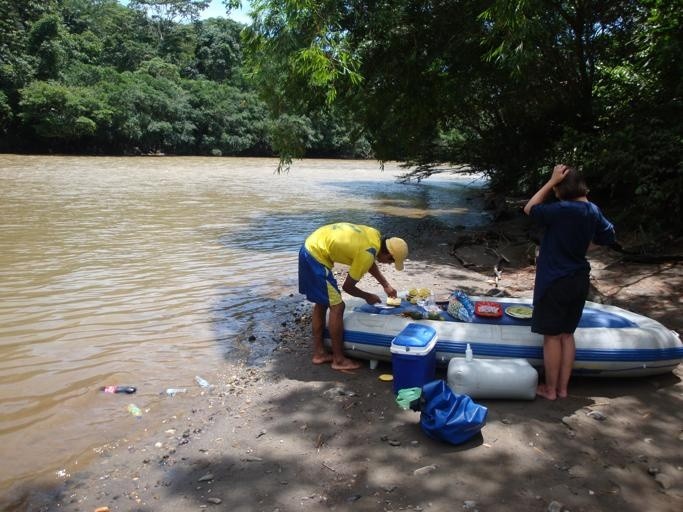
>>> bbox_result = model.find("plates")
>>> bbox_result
[373,302,395,310]
[505,305,534,321]
[473,302,502,319]
[430,292,454,303]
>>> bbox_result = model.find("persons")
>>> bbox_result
[298,222,408,370]
[524,164,615,400]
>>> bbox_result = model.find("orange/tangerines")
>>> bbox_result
[430,312,439,320]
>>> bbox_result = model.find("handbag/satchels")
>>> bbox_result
[419,379,489,445]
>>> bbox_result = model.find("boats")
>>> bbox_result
[322,296,682,378]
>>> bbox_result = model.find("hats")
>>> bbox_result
[386,236,407,272]
